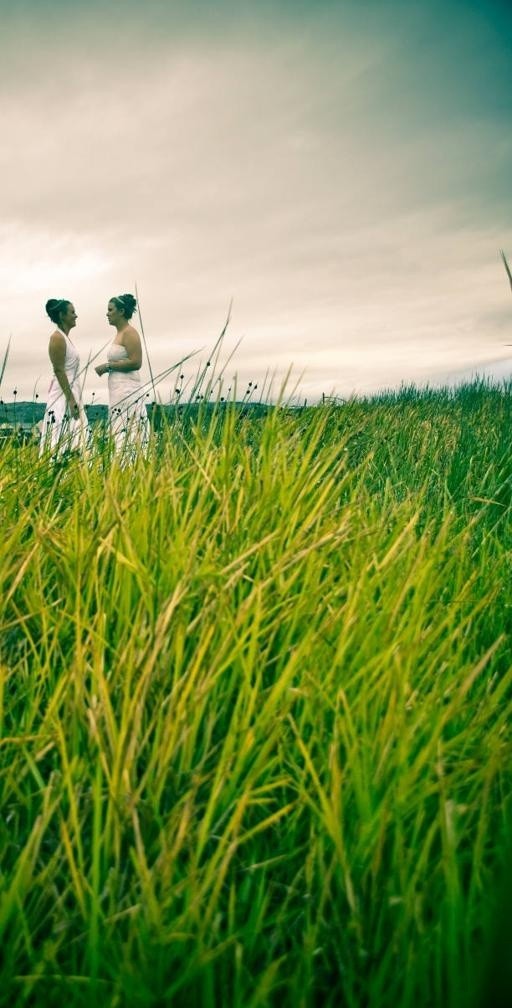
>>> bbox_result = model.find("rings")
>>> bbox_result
[100,369,102,371]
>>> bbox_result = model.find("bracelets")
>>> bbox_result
[106,363,111,372]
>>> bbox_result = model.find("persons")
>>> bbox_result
[38,299,96,467]
[94,294,151,472]
